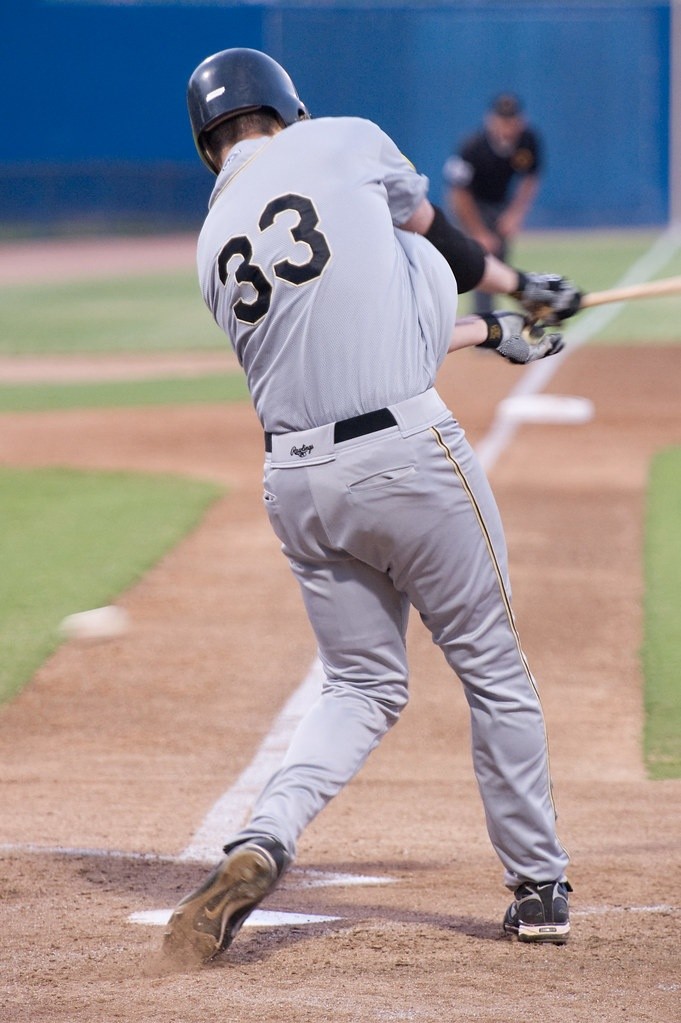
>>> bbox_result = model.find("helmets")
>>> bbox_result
[187,47,312,177]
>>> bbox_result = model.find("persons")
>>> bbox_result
[445,91,542,316]
[160,48,581,963]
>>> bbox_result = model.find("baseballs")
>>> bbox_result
[66,604,130,641]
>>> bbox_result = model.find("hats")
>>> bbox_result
[491,93,523,118]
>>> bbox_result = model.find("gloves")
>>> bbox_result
[474,310,566,366]
[508,271,583,323]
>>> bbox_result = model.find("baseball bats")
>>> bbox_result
[538,277,681,316]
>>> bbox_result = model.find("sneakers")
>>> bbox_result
[161,837,284,966]
[503,881,573,943]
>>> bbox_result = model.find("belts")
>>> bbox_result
[265,409,398,454]
